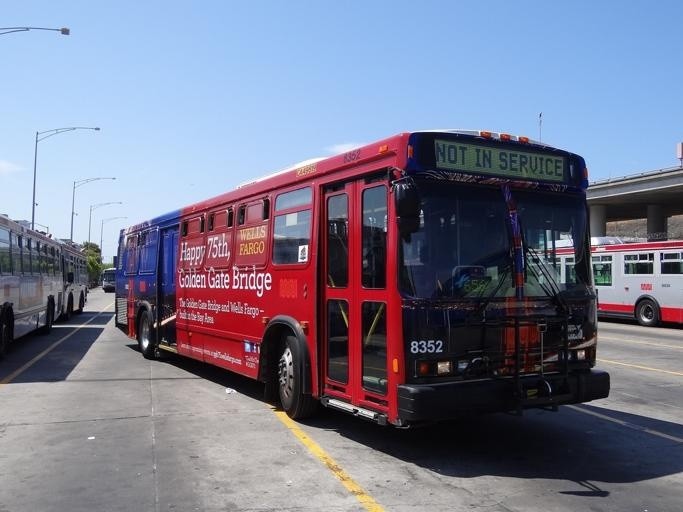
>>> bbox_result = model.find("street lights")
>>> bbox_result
[536,108,542,142]
[0,24,128,268]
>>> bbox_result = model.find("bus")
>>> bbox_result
[100,267,115,293]
[525,235,683,328]
[113,130,611,434]
[59,243,89,318]
[0,213,63,350]
